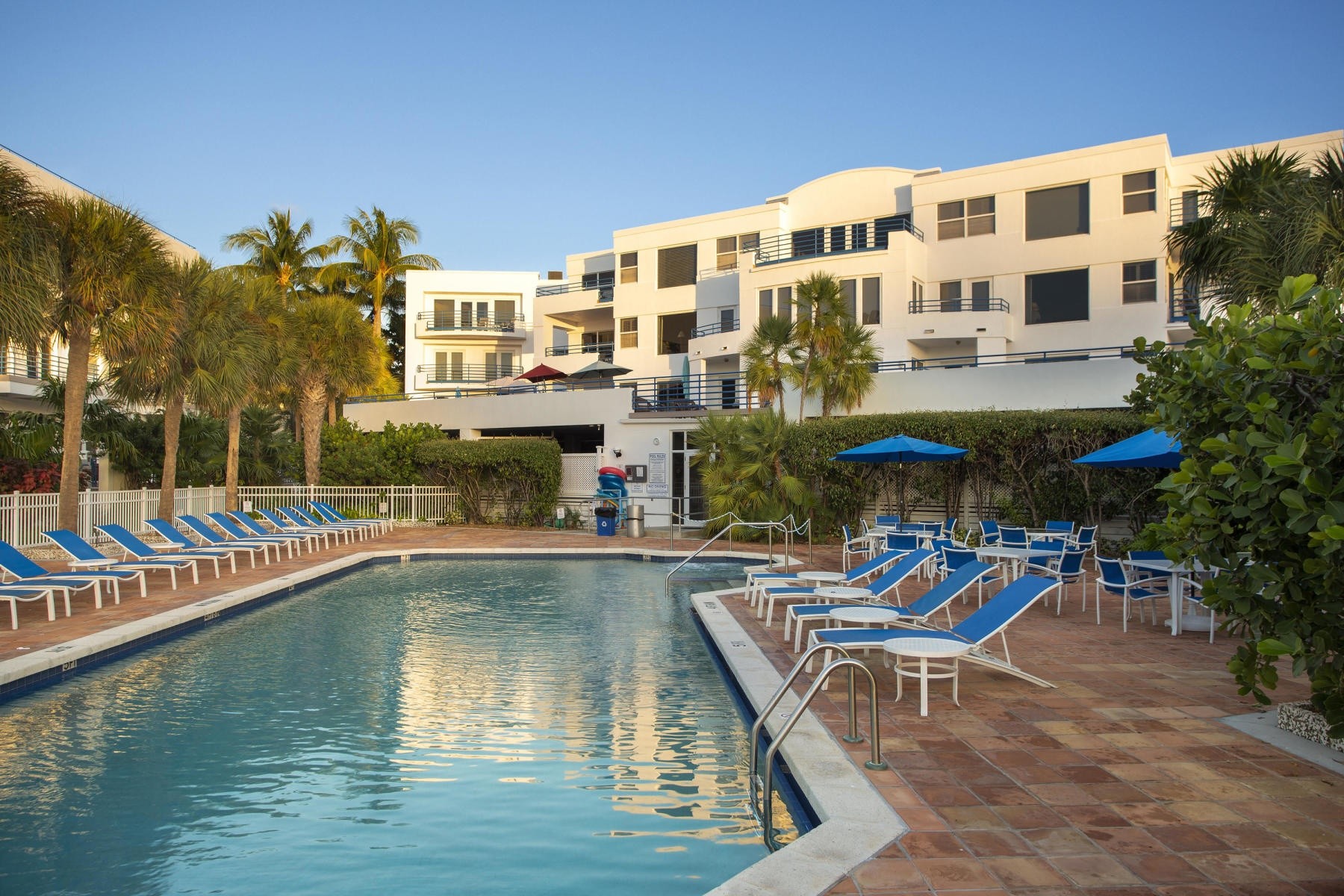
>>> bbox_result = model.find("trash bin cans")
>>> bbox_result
[595,507,616,536]
[626,505,645,538]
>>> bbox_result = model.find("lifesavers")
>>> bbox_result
[597,466,628,503]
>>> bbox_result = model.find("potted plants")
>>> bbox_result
[500,321,514,332]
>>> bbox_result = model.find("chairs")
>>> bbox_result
[634,397,707,418]
[478,317,492,331]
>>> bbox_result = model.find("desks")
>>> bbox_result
[876,522,938,530]
[796,571,848,588]
[813,587,874,607]
[884,636,970,716]
[971,546,1062,607]
[68,557,121,595]
[282,525,311,549]
[145,541,186,573]
[0,498,1287,692]
[830,607,899,673]
[1014,527,1073,545]
[869,530,935,578]
[1123,558,1239,633]
